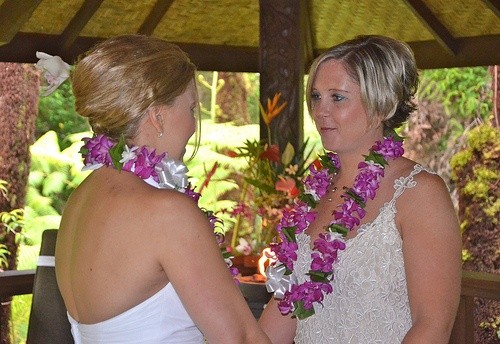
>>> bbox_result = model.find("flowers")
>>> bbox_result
[34,51,72,96]
[197,92,321,276]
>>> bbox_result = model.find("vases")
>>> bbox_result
[237,276,275,322]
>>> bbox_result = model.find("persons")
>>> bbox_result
[258,34,462,344]
[53,31,272,344]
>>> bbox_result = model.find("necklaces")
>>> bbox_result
[327,171,349,203]
